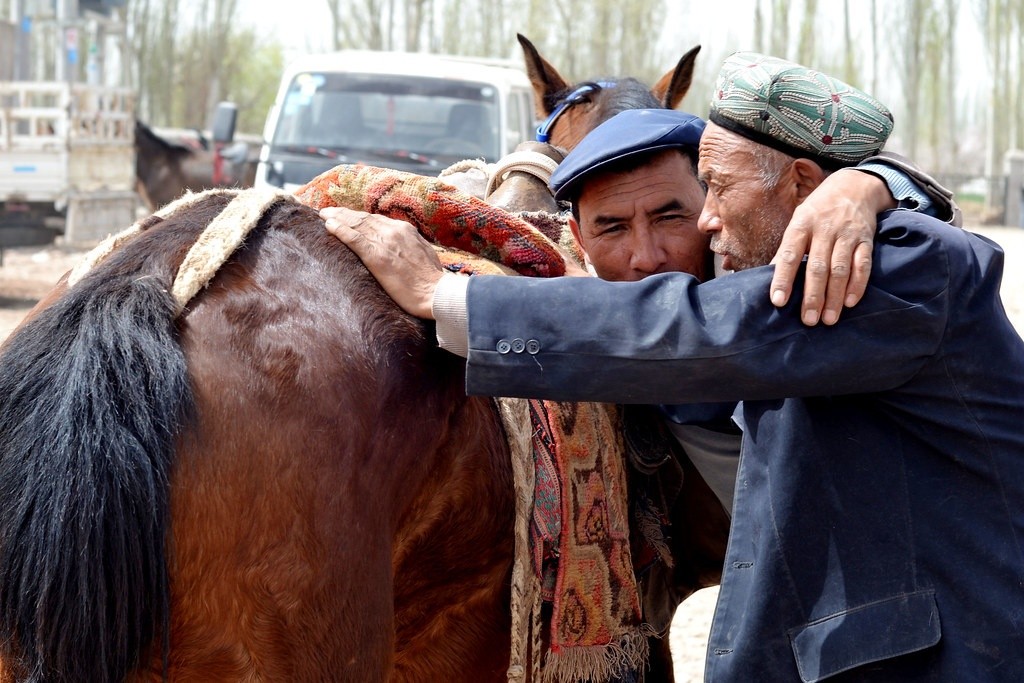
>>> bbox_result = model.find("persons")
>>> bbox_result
[315,48,1024,683]
[544,108,967,683]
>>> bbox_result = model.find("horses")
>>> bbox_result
[0,33,703,683]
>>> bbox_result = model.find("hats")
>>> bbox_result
[550,107,706,202]
[709,53,894,172]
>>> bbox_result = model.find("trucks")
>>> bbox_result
[0,81,141,247]
[208,49,556,216]
